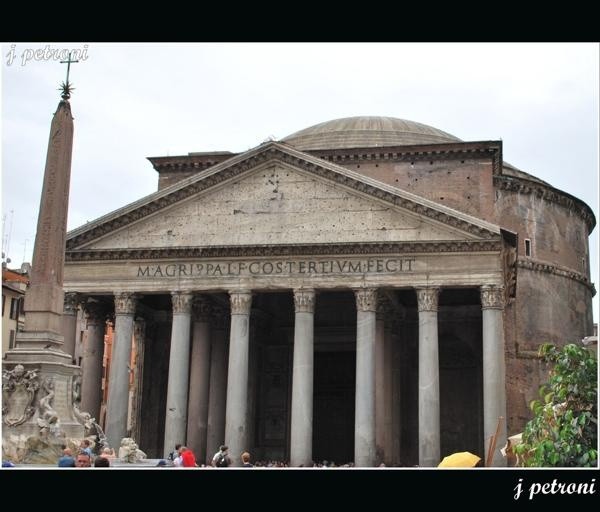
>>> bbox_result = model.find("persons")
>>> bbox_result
[57,440,109,467]
[154,444,354,468]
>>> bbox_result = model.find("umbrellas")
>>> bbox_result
[437,451,481,468]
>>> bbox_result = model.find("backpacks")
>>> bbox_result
[216,454,228,467]
